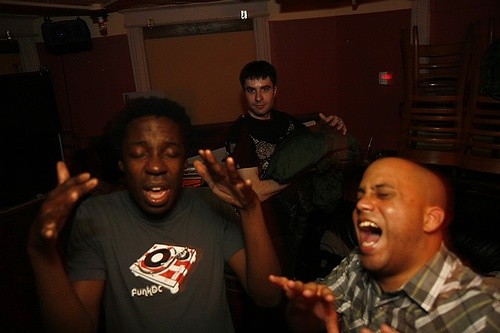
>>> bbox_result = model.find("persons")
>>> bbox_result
[265,156,499,333]
[24,93,285,333]
[222,58,352,287]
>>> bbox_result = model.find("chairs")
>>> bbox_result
[400,16,500,180]
